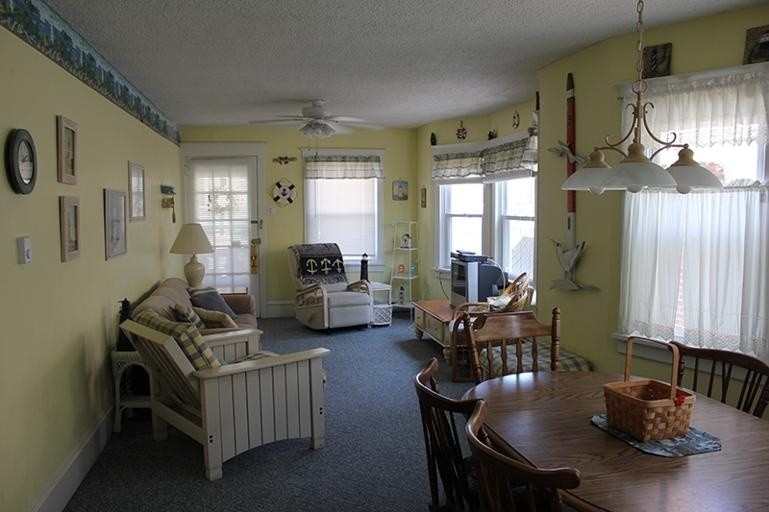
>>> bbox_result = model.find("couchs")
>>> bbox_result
[287,243,372,330]
[118,313,330,481]
[130,278,255,335]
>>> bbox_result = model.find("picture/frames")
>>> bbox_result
[128,159,146,221]
[103,187,128,261]
[420,189,426,208]
[57,116,81,186]
[392,181,409,200]
[743,24,769,65]
[58,195,80,264]
[643,43,671,78]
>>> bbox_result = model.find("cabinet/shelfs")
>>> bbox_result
[390,219,419,321]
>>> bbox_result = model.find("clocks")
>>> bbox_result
[5,129,38,195]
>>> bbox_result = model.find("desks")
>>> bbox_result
[412,298,480,363]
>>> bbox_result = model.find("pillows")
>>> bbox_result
[170,303,205,328]
[193,306,238,327]
[189,291,233,319]
[187,287,217,293]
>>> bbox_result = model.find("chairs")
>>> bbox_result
[458,307,562,382]
[450,272,529,381]
[460,400,581,512]
[412,356,484,512]
[668,342,768,419]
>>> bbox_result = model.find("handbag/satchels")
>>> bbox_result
[603,334,695,442]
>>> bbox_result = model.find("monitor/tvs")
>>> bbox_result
[450,258,509,311]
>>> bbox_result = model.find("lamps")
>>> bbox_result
[298,118,336,161]
[560,1,725,195]
[170,224,215,289]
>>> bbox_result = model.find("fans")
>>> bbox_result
[247,112,371,138]
